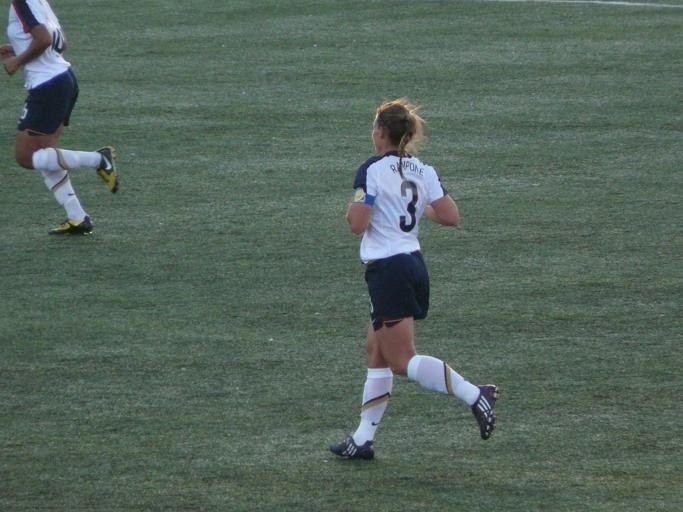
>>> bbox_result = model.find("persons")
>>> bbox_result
[0,0,118,235]
[330,102,499,459]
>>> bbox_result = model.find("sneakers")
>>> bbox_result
[47,213,93,236]
[474,384,498,440]
[330,436,374,460]
[96,145,118,194]
